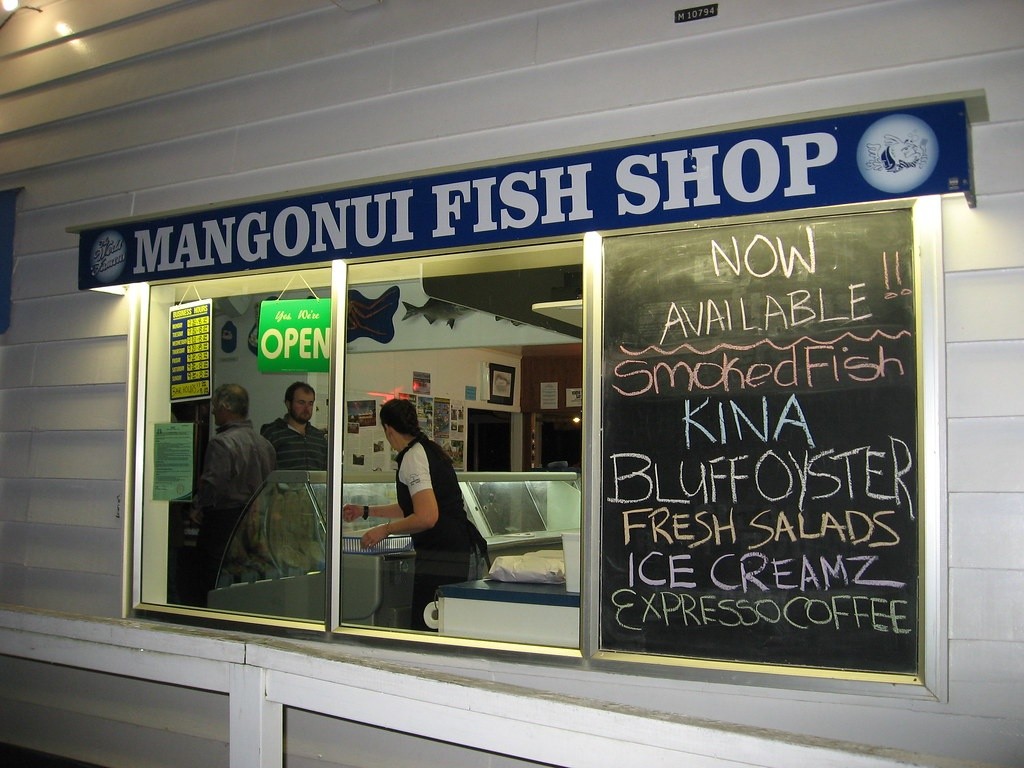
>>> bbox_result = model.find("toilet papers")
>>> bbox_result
[423,601,439,630]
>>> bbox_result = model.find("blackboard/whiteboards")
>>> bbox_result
[592,195,927,688]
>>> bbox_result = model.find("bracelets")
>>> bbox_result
[362,505,369,520]
[386,521,392,534]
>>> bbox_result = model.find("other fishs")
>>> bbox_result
[404,297,478,328]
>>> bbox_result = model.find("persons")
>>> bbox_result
[262,381,328,471]
[168,412,187,605]
[193,383,276,608]
[343,399,491,633]
